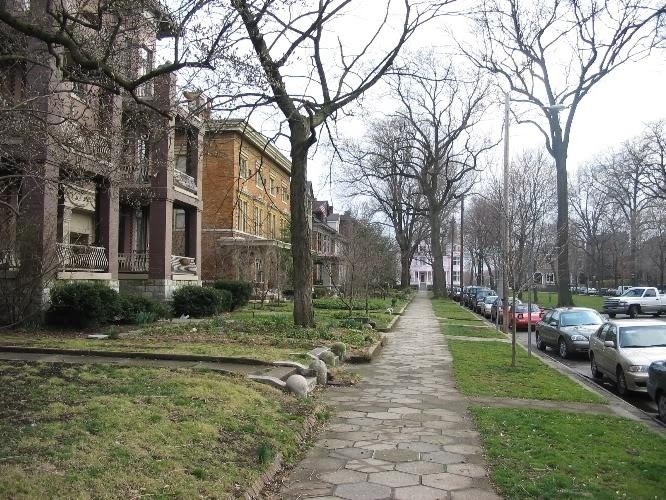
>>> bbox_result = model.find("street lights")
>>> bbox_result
[502,105,565,334]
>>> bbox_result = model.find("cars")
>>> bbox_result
[453,285,543,332]
[536,306,608,359]
[569,286,633,296]
[588,319,666,422]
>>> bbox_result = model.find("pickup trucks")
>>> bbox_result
[602,287,666,319]
